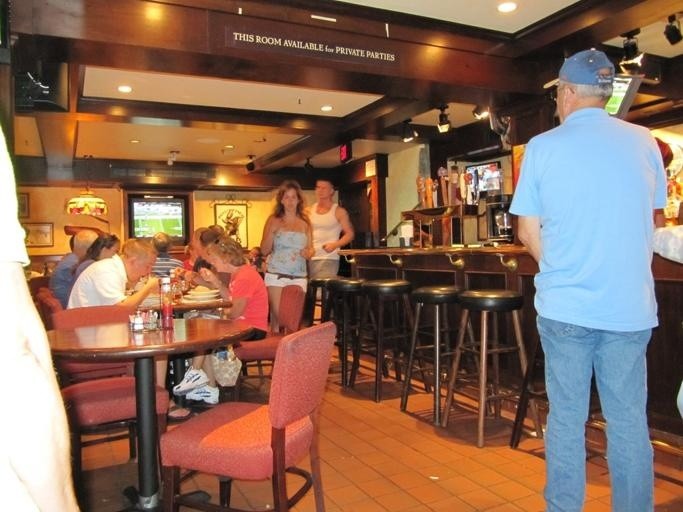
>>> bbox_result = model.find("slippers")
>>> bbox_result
[165,400,192,420]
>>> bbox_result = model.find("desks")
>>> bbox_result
[144,300,242,408]
[400,285,492,425]
[45,318,255,511]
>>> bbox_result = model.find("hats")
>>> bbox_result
[542,48,614,90]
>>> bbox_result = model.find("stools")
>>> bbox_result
[311,275,388,386]
[510,332,548,449]
[442,290,543,449]
[349,279,431,402]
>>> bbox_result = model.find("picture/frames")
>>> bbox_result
[17,192,30,218]
[21,222,54,247]
[215,203,248,250]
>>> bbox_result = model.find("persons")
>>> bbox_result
[148,233,183,276]
[483,163,500,189]
[508,48,667,512]
[172,241,269,403]
[245,247,266,273]
[47,226,119,307]
[246,181,315,335]
[304,178,355,325]
[67,239,192,421]
[183,224,231,290]
[0,131,80,511]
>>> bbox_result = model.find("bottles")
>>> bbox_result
[128,307,157,331]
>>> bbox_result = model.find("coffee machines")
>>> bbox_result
[487,195,514,243]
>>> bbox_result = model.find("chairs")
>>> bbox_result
[51,306,168,483]
[160,321,336,511]
[233,285,305,402]
[36,287,130,387]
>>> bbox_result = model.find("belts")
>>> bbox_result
[270,272,310,281]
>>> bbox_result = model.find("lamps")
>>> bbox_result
[621,14,682,60]
[400,102,492,144]
[63,155,108,214]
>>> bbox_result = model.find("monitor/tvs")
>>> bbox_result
[129,198,185,243]
[465,160,501,202]
[16,62,70,113]
[605,73,646,119]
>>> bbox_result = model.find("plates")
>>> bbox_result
[182,289,223,304]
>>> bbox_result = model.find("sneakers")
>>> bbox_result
[171,364,210,395]
[185,384,219,406]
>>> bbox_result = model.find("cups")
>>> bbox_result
[161,268,190,301]
[162,299,175,329]
